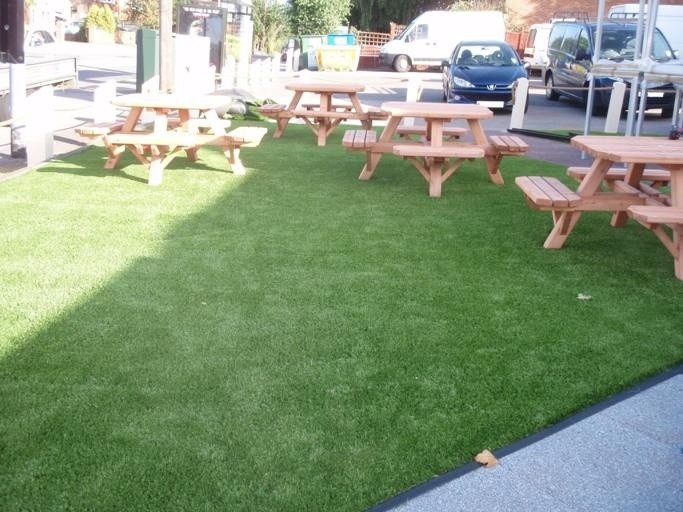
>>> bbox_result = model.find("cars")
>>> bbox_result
[439,39,531,114]
[25,10,127,61]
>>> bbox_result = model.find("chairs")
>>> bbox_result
[457,50,506,65]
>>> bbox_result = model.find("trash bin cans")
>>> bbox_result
[299,34,361,72]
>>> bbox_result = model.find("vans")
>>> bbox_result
[378,8,506,73]
[523,2,682,119]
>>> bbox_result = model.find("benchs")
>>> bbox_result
[257,102,387,147]
[342,125,530,200]
[513,166,683,280]
[74,118,268,188]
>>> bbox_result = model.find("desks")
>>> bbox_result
[102,92,244,187]
[272,80,371,147]
[544,134,682,278]
[357,101,505,198]
